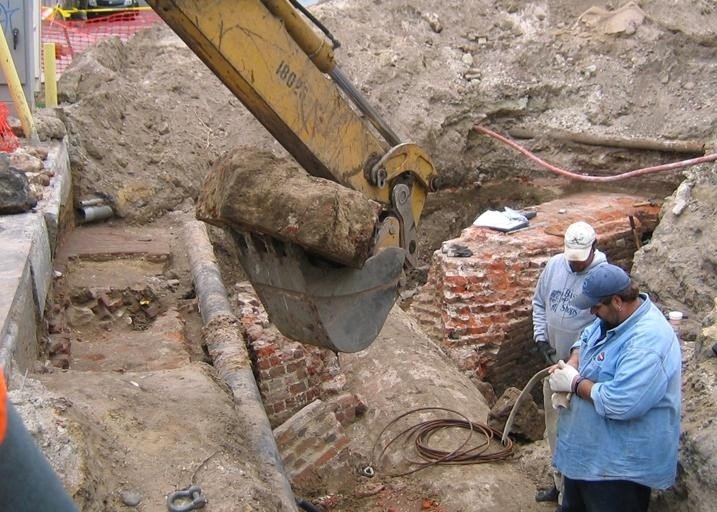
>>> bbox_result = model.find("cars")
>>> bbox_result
[87,0,139,21]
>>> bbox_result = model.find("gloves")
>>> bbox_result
[535,341,556,365]
[548,359,578,410]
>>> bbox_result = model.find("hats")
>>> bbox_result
[572,263,631,309]
[564,222,596,261]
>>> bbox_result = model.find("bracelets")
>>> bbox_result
[575,377,588,399]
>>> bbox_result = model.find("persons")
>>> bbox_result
[532,221,607,512]
[548,263,682,512]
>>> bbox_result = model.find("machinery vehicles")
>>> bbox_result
[144,0,449,356]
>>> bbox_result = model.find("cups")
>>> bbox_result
[668,311,682,335]
